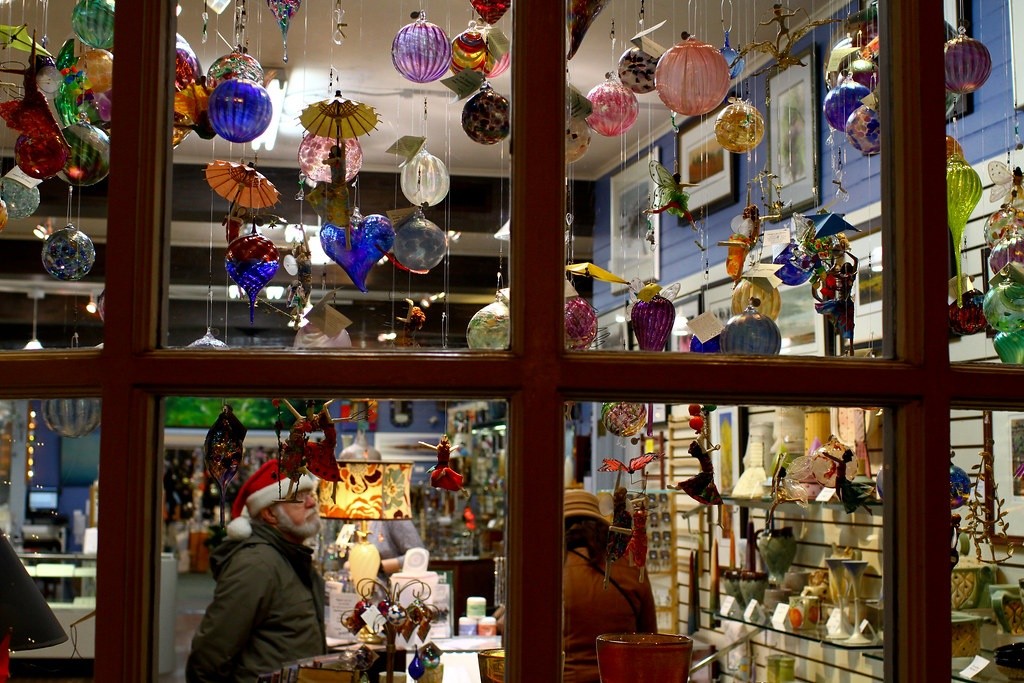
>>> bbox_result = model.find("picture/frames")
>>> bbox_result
[841,227,882,355]
[703,271,748,328]
[981,252,1001,338]
[666,294,703,355]
[628,320,668,352]
[859,1,973,123]
[984,410,1024,548]
[593,300,630,351]
[765,43,821,225]
[759,255,833,357]
[705,403,748,574]
[609,146,661,295]
[676,95,740,229]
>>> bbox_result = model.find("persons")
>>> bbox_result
[343,519,428,581]
[563,489,658,683]
[184,458,328,683]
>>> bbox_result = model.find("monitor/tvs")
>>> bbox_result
[29,492,58,511]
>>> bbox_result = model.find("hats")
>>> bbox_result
[225,458,315,542]
[563,489,611,526]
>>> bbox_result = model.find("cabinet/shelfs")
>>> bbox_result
[8,554,177,678]
[450,401,584,557]
[718,495,880,651]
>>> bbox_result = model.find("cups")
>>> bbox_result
[788,596,820,630]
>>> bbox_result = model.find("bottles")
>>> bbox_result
[596,634,692,683]
[725,569,767,610]
[478,648,565,683]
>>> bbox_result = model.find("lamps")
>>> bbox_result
[316,462,412,599]
